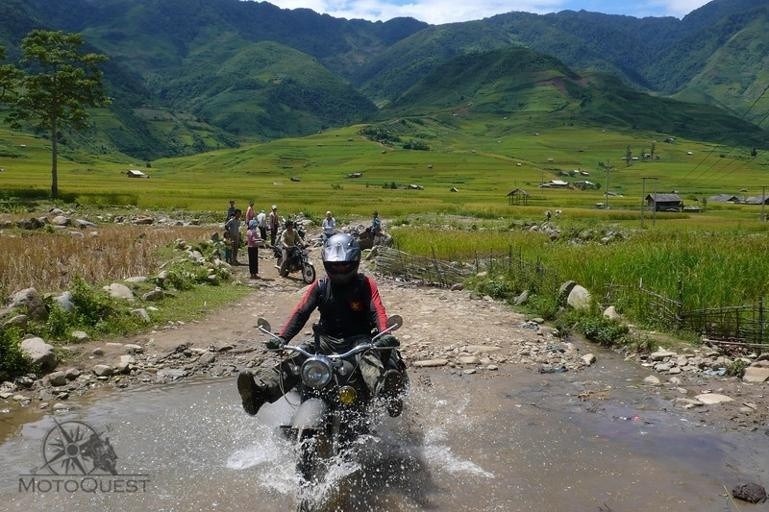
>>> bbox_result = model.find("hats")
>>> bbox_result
[249,205,333,227]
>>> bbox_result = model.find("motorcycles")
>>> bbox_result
[253,315,406,512]
[272,242,318,283]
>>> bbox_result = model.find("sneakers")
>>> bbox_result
[225,245,275,279]
[237,371,264,417]
[384,371,404,416]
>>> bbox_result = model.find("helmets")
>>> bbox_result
[320,232,362,263]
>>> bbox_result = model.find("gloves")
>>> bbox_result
[266,336,285,353]
[380,334,400,348]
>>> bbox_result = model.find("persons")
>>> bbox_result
[236,232,408,418]
[223,199,383,278]
